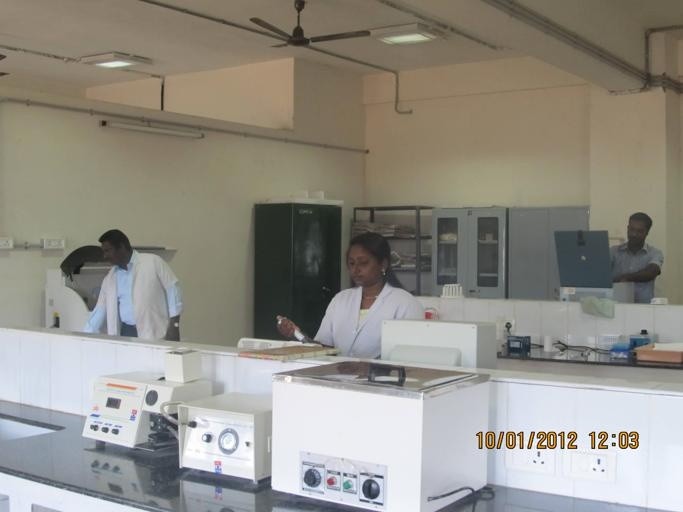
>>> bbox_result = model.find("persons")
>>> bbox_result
[83,230,184,341]
[608,213,662,303]
[275,232,423,360]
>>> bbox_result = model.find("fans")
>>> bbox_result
[249,0,371,48]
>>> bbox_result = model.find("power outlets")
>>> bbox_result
[0,237,14,251]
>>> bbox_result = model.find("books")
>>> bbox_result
[237,346,338,361]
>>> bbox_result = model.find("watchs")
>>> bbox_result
[169,321,179,329]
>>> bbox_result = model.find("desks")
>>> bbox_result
[498,342,683,369]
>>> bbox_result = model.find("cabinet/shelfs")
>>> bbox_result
[253,203,342,343]
[431,207,589,300]
[350,205,436,296]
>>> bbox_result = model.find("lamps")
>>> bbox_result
[102,121,204,140]
[371,24,442,46]
